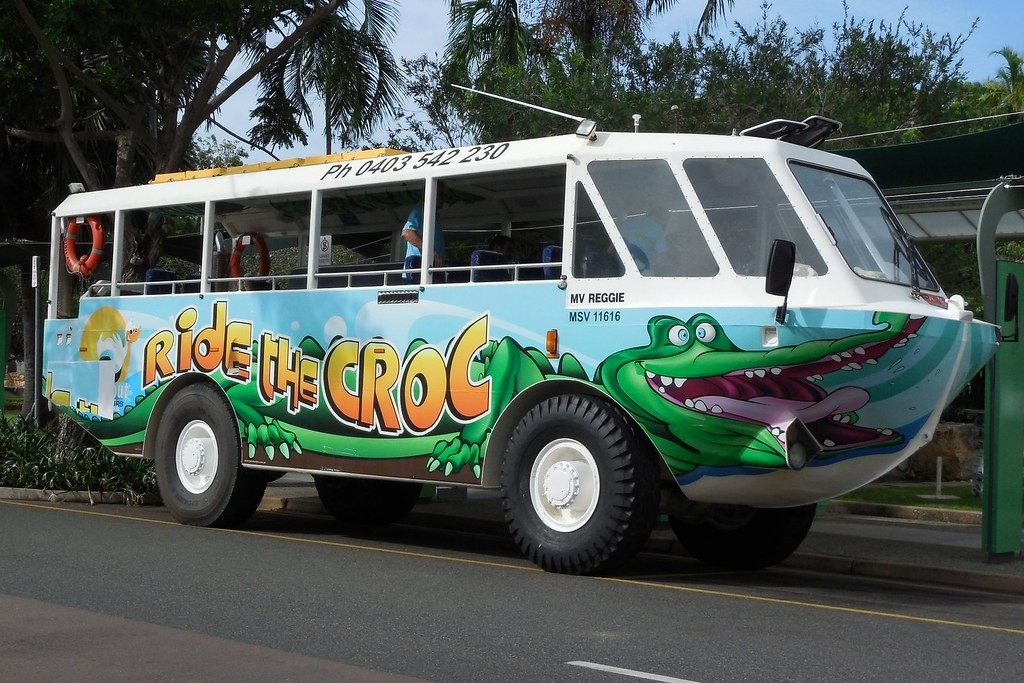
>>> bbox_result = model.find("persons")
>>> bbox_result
[401,180,446,284]
[479,235,622,282]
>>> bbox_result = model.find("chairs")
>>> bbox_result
[143,244,621,294]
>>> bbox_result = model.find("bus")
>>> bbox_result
[40,116,1003,578]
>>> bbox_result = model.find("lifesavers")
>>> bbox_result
[62,215,105,277]
[228,230,272,287]
